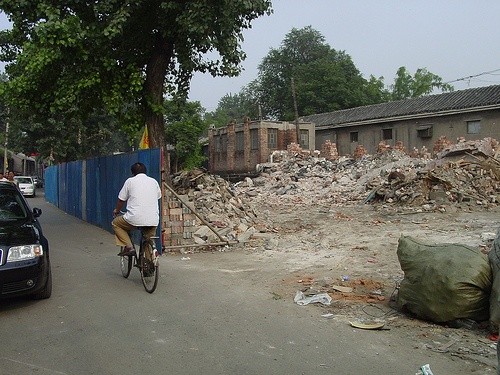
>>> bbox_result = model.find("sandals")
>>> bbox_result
[118,246,135,256]
[143,270,153,278]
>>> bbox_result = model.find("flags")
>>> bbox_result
[137,126,149,149]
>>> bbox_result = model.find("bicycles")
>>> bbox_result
[113,210,160,294]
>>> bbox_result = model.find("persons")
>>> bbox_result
[111,162,162,278]
[0,171,18,186]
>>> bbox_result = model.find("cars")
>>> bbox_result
[12,176,44,197]
[0,180,53,301]
